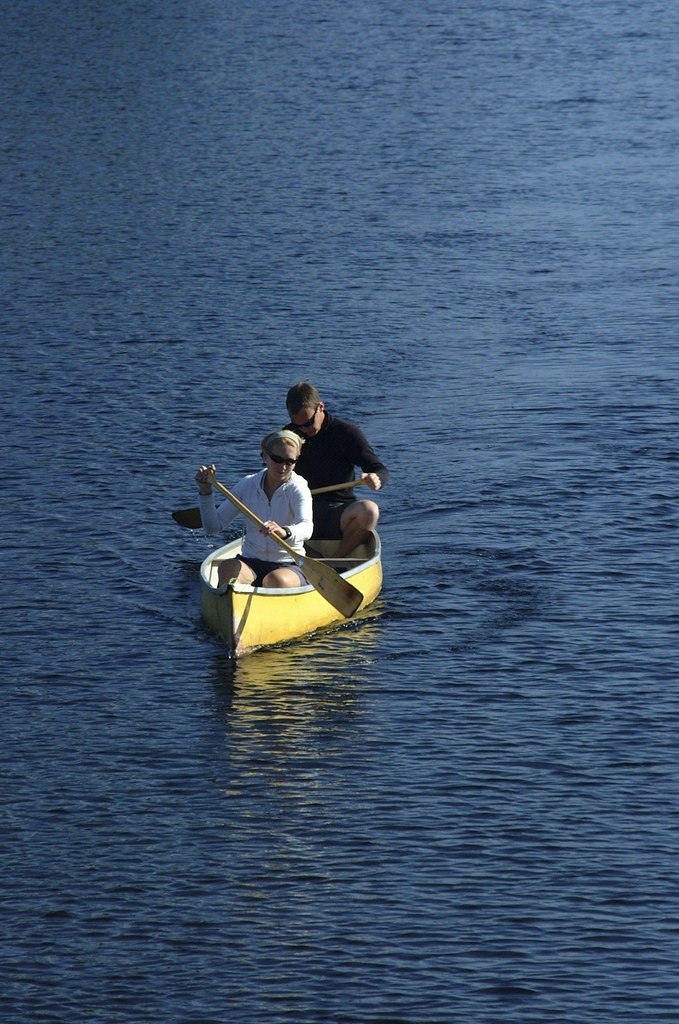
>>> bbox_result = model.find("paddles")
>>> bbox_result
[171,477,368,530]
[204,467,366,619]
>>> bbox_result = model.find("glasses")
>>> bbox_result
[291,403,320,427]
[267,450,297,466]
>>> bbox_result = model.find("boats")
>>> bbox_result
[196,524,384,657]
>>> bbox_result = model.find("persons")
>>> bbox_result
[281,382,388,558]
[195,430,314,588]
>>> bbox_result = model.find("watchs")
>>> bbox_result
[281,527,292,540]
